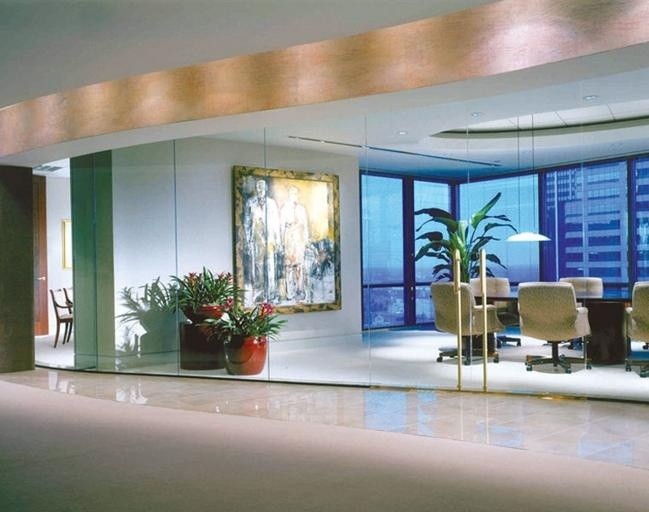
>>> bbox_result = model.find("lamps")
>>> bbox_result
[505,117,554,245]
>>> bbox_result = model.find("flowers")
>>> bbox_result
[157,266,243,313]
[202,303,287,348]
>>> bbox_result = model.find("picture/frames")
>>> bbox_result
[230,164,343,312]
[61,218,72,269]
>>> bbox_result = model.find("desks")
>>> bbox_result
[462,289,635,365]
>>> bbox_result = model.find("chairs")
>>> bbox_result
[517,280,592,374]
[624,282,648,379]
[49,288,74,348]
[469,276,521,348]
[559,275,603,349]
[429,282,505,366]
[62,287,74,344]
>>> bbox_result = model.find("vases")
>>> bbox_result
[220,336,268,374]
[179,305,227,321]
